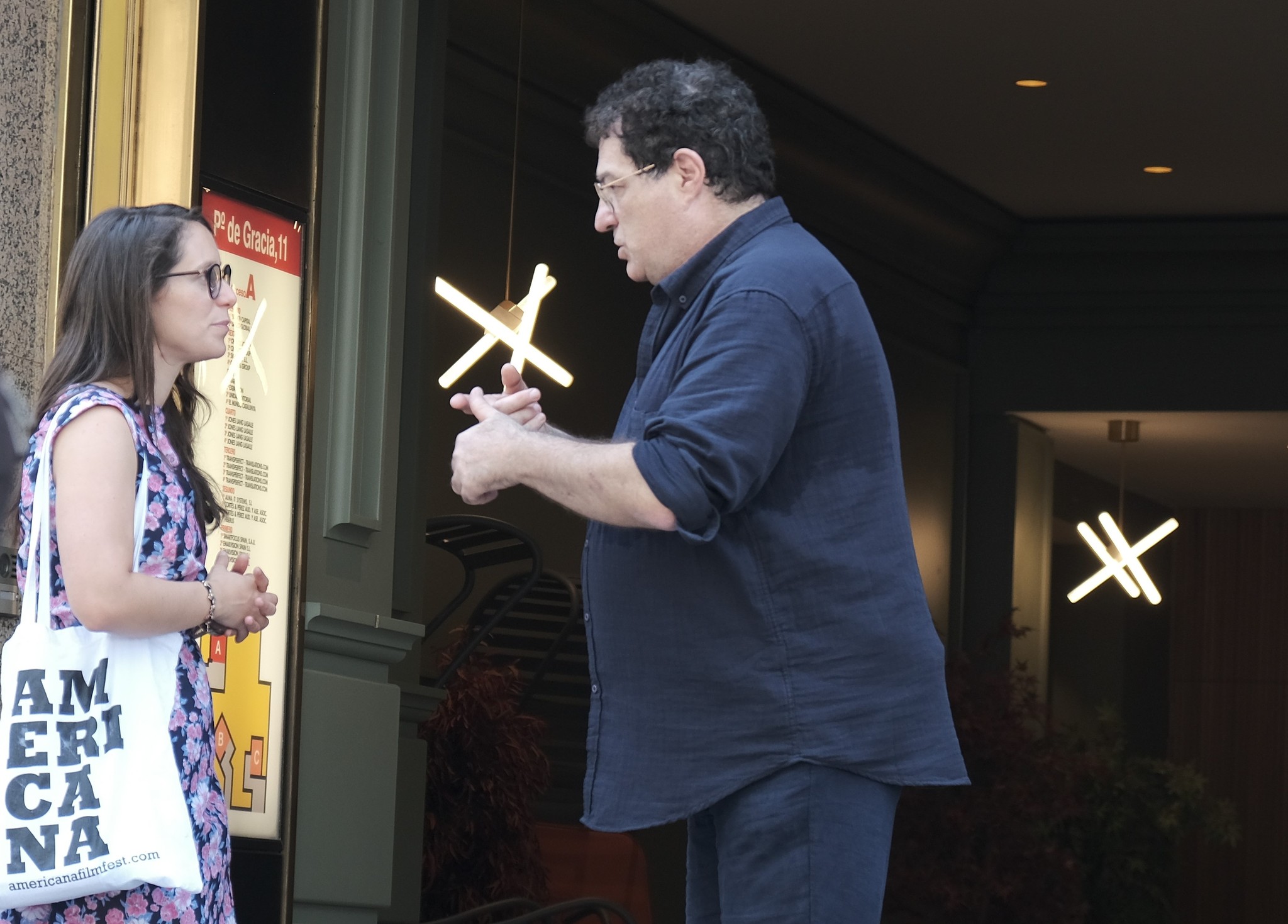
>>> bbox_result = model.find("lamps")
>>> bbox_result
[435,1,573,389]
[1066,420,1179,606]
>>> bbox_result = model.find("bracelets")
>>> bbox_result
[200,581,218,632]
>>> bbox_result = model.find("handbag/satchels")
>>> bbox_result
[0,613,208,909]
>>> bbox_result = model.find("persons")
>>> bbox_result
[1,195,279,924]
[445,55,970,923]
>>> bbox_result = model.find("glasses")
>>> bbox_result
[155,263,231,300]
[592,162,656,205]
